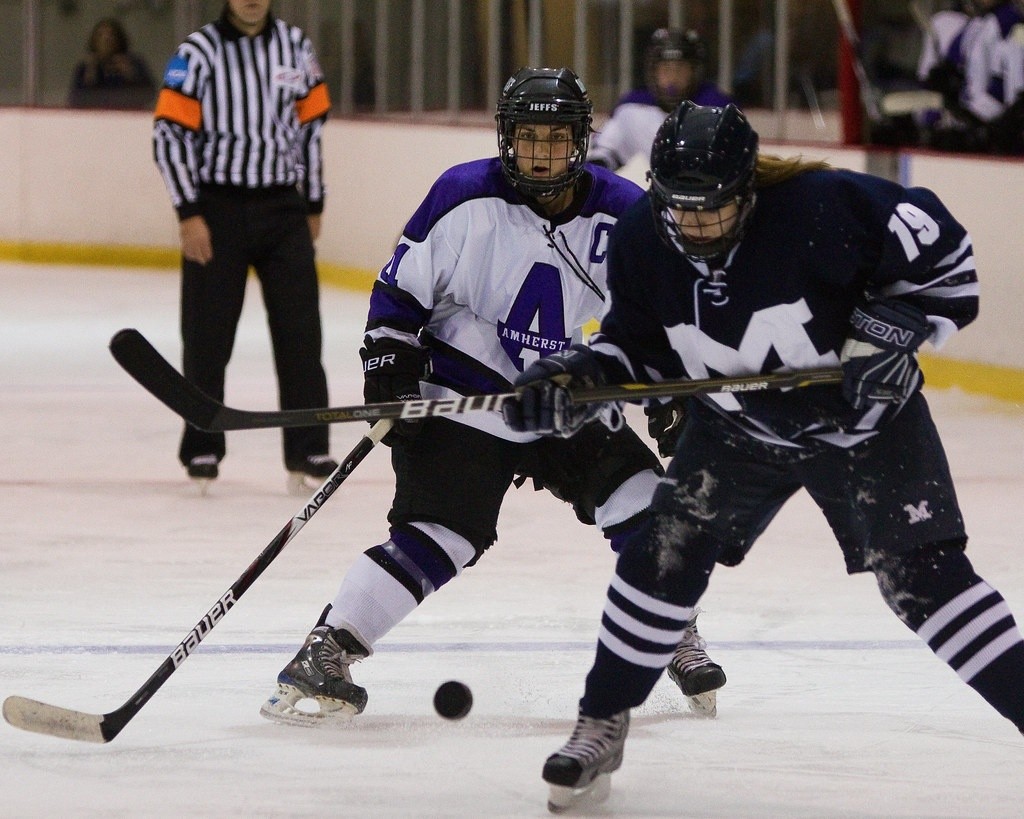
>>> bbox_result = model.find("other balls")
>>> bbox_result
[434,680,473,721]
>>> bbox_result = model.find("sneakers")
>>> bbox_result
[541,699,630,813]
[286,452,336,494]
[261,603,368,728]
[666,618,725,716]
[189,456,219,497]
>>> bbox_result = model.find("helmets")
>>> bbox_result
[645,26,701,100]
[646,101,758,261]
[496,63,592,197]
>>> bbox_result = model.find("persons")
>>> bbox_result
[501,99,1024,813]
[585,26,731,176]
[148,0,340,490]
[62,19,156,113]
[914,0,1024,157]
[257,64,726,723]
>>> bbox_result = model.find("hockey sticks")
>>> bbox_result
[109,326,844,432]
[3,417,394,742]
[835,0,945,121]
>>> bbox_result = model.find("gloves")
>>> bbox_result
[360,332,423,450]
[499,342,612,438]
[832,300,929,410]
